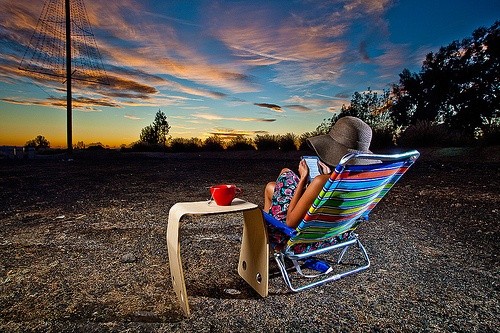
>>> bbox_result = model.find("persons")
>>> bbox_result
[264,115,374,255]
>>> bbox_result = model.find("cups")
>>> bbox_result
[209,184,241,206]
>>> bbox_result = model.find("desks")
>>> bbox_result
[166,196,269,317]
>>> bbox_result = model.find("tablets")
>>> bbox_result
[302,154,322,185]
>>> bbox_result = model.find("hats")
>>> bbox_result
[306,116,383,167]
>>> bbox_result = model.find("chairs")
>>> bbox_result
[260,150,421,295]
